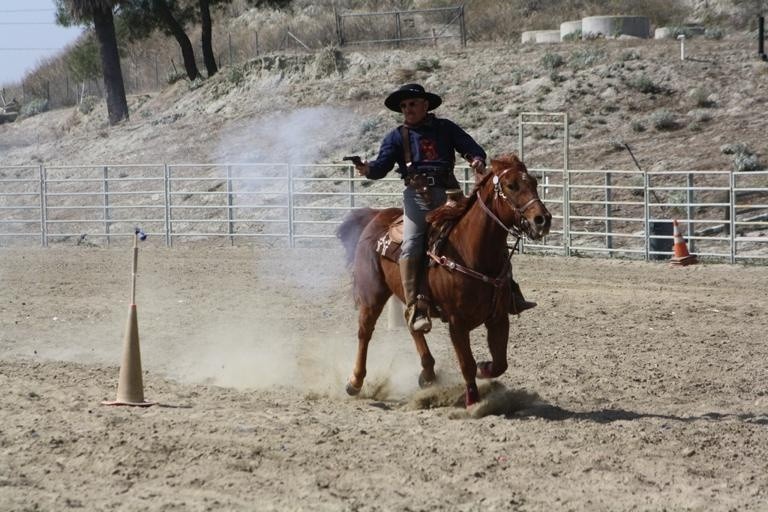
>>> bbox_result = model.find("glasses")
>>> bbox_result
[400,102,415,109]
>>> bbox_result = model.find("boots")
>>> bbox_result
[399,258,432,331]
[507,285,537,316]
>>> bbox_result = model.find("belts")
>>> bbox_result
[403,174,457,187]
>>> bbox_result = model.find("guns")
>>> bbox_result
[343,156,364,168]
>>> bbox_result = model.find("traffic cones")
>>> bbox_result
[669,219,698,267]
[100,305,153,406]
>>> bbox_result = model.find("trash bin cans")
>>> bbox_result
[648,223,674,260]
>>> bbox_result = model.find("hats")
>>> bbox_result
[385,83,442,113]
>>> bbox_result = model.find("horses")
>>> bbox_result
[332,152,552,417]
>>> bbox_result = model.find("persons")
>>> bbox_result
[354,83,538,332]
